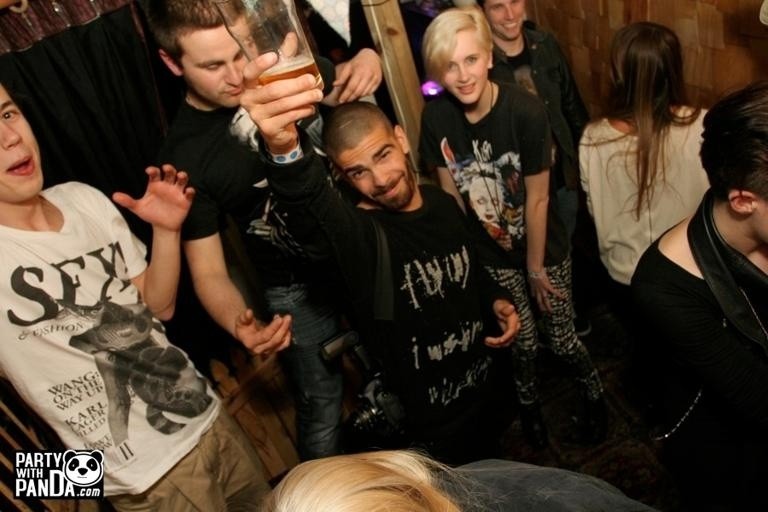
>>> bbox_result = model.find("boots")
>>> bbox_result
[585,393,610,442]
[523,402,548,452]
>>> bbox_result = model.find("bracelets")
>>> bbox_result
[526,269,548,279]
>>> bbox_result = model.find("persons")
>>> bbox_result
[577,23,709,407]
[476,0,596,338]
[239,33,522,470]
[627,82,767,511]
[256,447,655,510]
[420,8,613,465]
[146,2,387,461]
[0,82,273,511]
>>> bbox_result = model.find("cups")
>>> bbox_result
[215,1,326,104]
